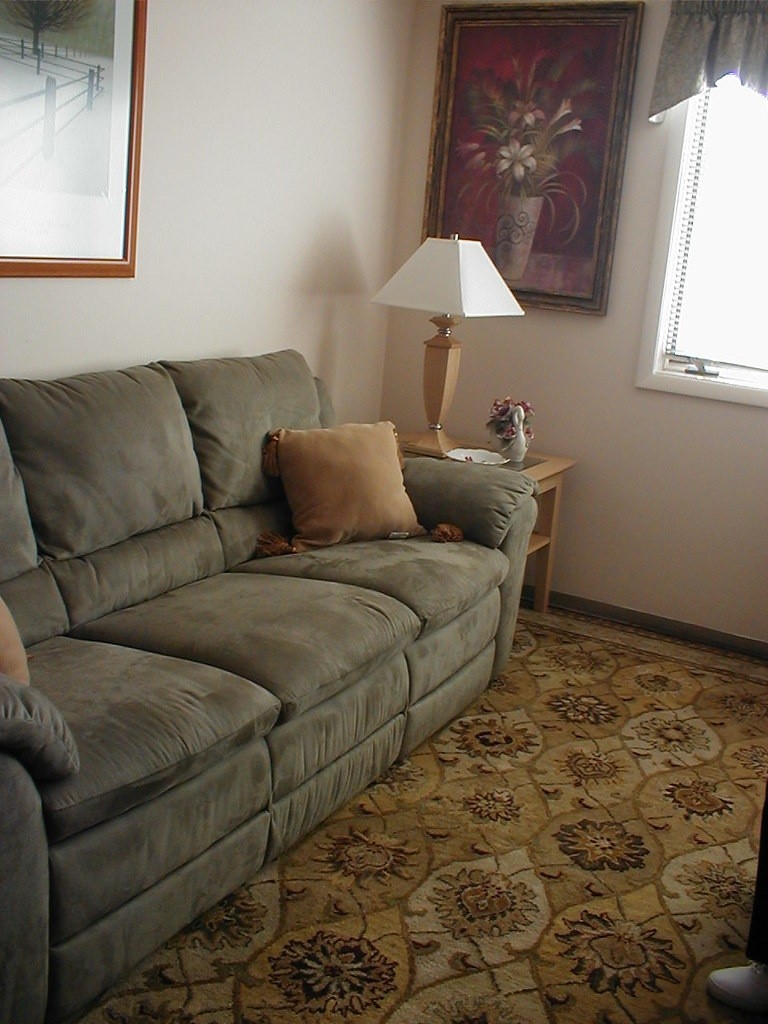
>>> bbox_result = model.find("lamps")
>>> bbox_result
[369,231,524,457]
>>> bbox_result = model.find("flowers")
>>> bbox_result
[480,396,538,451]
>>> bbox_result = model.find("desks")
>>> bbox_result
[402,441,578,612]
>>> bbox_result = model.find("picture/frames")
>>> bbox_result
[0,1,149,278]
[418,0,649,318]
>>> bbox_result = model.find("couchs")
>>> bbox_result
[0,345,537,1024]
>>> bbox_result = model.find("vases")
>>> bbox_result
[488,405,530,463]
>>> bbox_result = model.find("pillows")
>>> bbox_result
[256,419,465,559]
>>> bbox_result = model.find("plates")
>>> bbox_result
[446,448,510,465]
